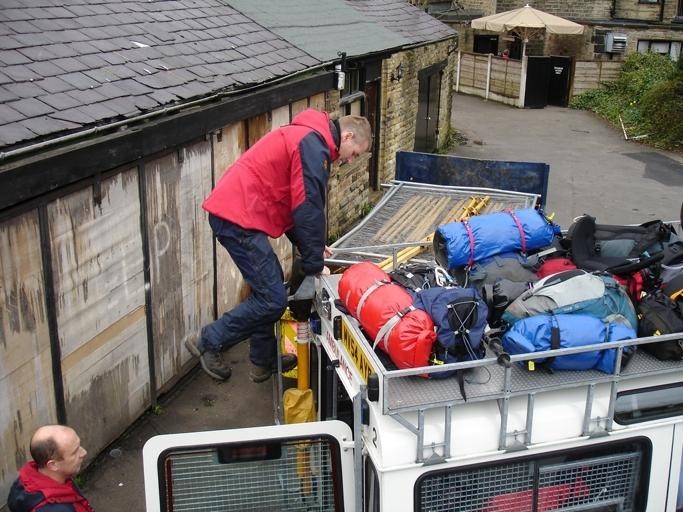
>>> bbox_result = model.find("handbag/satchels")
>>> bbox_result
[338,208,683,377]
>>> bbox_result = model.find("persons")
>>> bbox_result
[8,424,93,512]
[185,114,373,381]
[501,49,511,59]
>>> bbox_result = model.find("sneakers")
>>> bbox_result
[184,328,231,380]
[249,352,297,383]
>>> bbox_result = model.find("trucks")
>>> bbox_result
[138,215,682,512]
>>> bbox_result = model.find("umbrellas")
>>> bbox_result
[472,3,584,61]
